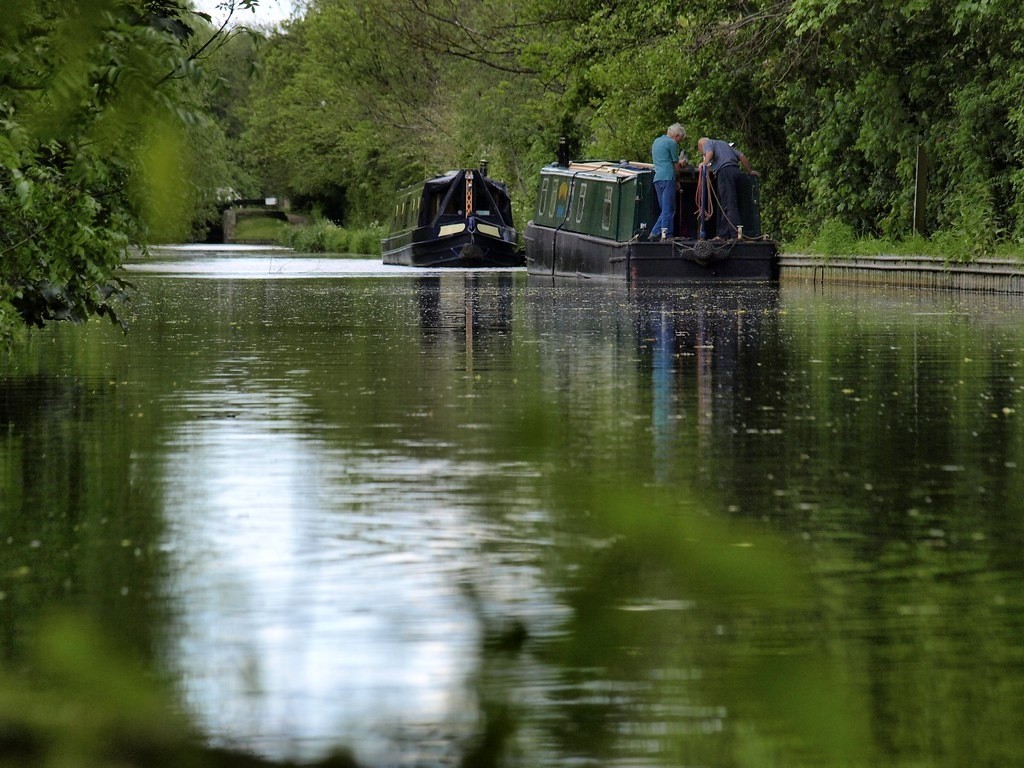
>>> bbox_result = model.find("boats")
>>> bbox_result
[379,156,524,268]
[522,115,783,289]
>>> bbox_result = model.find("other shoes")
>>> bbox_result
[649,235,660,242]
[708,236,721,241]
[661,237,674,243]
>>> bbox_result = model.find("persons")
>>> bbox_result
[648,122,687,243]
[698,137,759,242]
